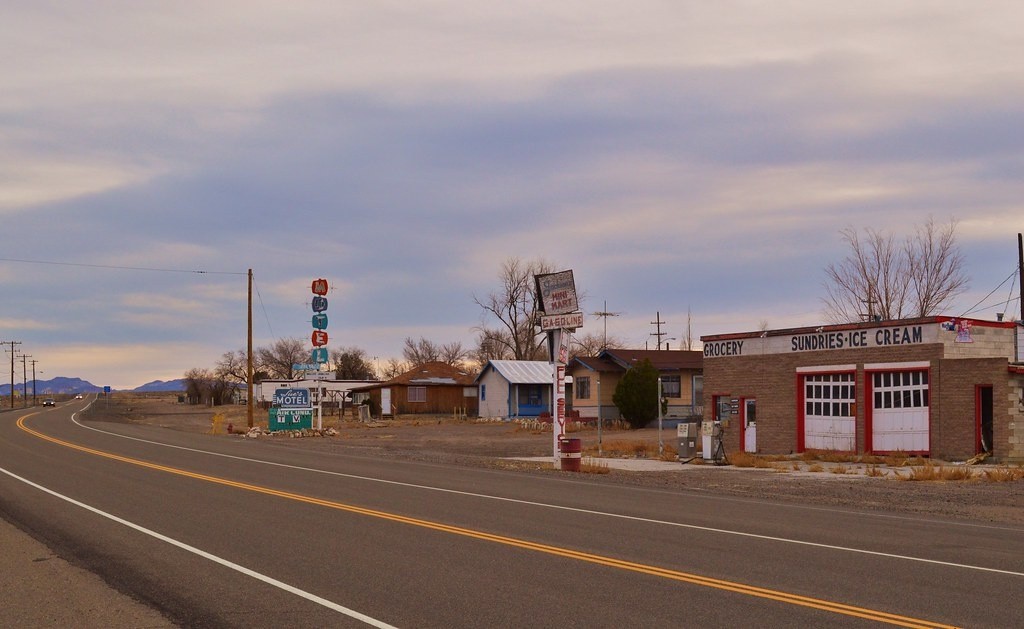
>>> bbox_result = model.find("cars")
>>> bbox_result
[43,398,55,407]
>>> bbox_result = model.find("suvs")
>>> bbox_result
[76,394,83,400]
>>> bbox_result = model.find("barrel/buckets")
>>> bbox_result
[561,439,581,473]
